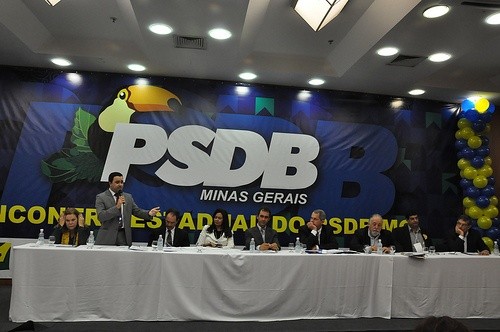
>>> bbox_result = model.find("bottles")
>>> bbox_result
[38,229,44,246]
[89,231,94,248]
[493,241,499,254]
[377,239,382,255]
[295,237,300,253]
[250,238,255,252]
[157,235,163,251]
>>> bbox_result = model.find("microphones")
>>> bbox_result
[119,190,126,205]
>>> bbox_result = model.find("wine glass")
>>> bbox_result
[301,244,307,254]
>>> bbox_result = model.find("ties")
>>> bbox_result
[166,230,172,247]
[114,193,124,232]
[316,232,320,249]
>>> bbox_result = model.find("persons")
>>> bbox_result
[449,215,491,255]
[148,208,190,247]
[53,209,90,245]
[396,211,433,252]
[196,209,234,248]
[299,210,338,249]
[415,316,472,332]
[94,172,161,246]
[244,207,281,250]
[350,214,400,254]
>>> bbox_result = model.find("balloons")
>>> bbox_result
[456,97,500,252]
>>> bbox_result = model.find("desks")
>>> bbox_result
[8,243,500,323]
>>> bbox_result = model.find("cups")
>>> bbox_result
[363,245,369,253]
[151,241,158,251]
[423,247,428,255]
[429,246,435,254]
[390,245,396,254]
[196,243,203,253]
[49,236,55,246]
[289,243,294,251]
